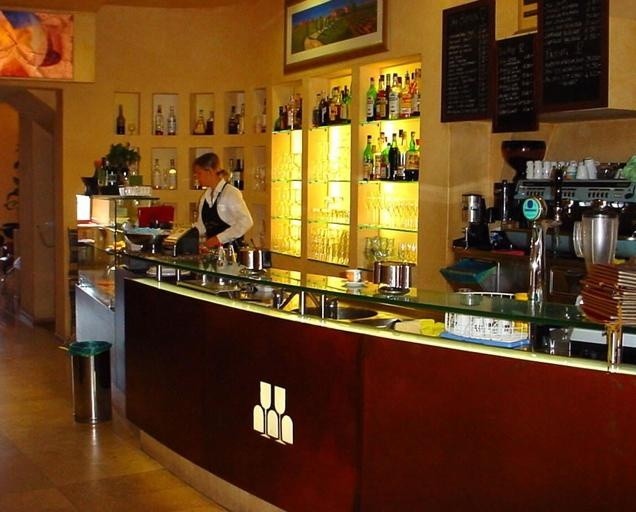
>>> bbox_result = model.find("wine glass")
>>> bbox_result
[365,196,421,227]
[274,189,301,219]
[274,225,300,256]
[364,236,418,265]
[162,167,168,190]
[253,168,266,191]
[127,121,135,135]
[276,153,301,180]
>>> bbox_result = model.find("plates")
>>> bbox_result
[124,232,169,251]
[342,278,368,287]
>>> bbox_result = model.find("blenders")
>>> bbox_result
[447,191,493,251]
[571,207,622,308]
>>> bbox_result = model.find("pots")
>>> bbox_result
[236,247,272,271]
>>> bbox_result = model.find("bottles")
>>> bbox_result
[365,70,421,120]
[275,93,302,129]
[313,83,352,126]
[116,104,125,135]
[152,158,162,189]
[168,159,177,189]
[98,156,130,197]
[216,245,224,261]
[192,158,244,190]
[227,245,234,258]
[362,130,420,181]
[153,101,267,136]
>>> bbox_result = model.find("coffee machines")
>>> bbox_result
[502,177,636,260]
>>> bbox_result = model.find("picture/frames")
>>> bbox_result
[283,0,391,73]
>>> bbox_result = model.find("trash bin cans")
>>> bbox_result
[70,341,111,424]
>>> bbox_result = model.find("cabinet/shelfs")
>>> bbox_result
[447,244,584,316]
[308,121,354,268]
[270,126,302,259]
[359,112,420,277]
[74,190,155,297]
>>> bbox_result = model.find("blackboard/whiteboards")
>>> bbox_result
[440,0,608,134]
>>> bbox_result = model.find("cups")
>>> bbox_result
[317,194,352,216]
[308,229,349,261]
[345,268,363,282]
[525,158,626,179]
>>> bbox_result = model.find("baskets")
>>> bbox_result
[444,292,531,342]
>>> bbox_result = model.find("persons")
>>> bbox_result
[186,151,253,252]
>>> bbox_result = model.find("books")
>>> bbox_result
[579,262,636,323]
